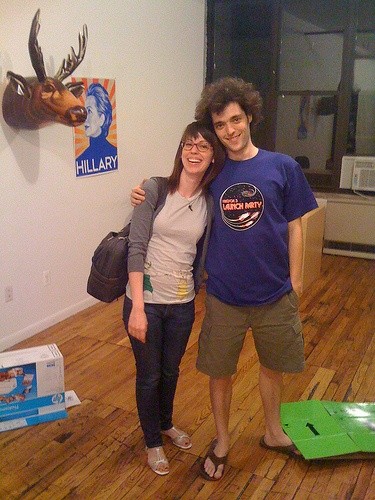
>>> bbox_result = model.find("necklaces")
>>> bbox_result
[177,185,202,211]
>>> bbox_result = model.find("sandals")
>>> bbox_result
[146,447,169,475]
[160,425,192,449]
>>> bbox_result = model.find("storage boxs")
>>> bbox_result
[0,343,82,432]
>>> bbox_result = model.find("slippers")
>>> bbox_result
[200,439,227,480]
[260,435,312,465]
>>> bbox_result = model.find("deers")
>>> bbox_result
[1,6,88,126]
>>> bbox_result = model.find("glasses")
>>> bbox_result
[181,139,210,153]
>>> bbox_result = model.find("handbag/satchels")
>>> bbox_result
[86,176,169,303]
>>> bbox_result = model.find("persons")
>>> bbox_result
[130,75,319,481]
[122,121,227,475]
